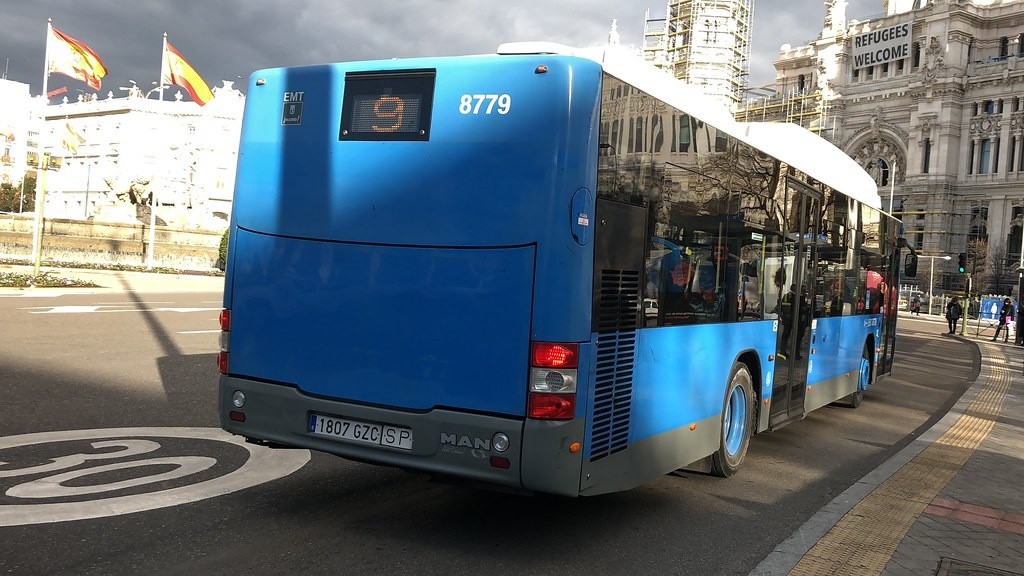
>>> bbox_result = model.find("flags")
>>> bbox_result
[164,41,215,106]
[49,25,109,90]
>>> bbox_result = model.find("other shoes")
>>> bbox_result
[991,339,995,341]
[1004,338,1008,343]
[779,351,789,359]
[795,354,802,361]
[949,331,955,334]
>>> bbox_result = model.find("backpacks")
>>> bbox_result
[950,302,959,318]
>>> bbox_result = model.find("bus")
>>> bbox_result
[216,37,919,498]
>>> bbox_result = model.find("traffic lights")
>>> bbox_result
[957,251,967,274]
[971,253,985,276]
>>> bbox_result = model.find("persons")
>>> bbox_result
[946,297,962,334]
[780,284,810,360]
[971,298,978,318]
[991,298,1015,343]
[1018,301,1024,346]
[908,257,916,276]
[827,281,885,315]
[911,294,921,316]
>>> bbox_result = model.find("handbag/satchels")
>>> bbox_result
[1007,323,1015,336]
[915,302,920,307]
[999,316,1005,321]
